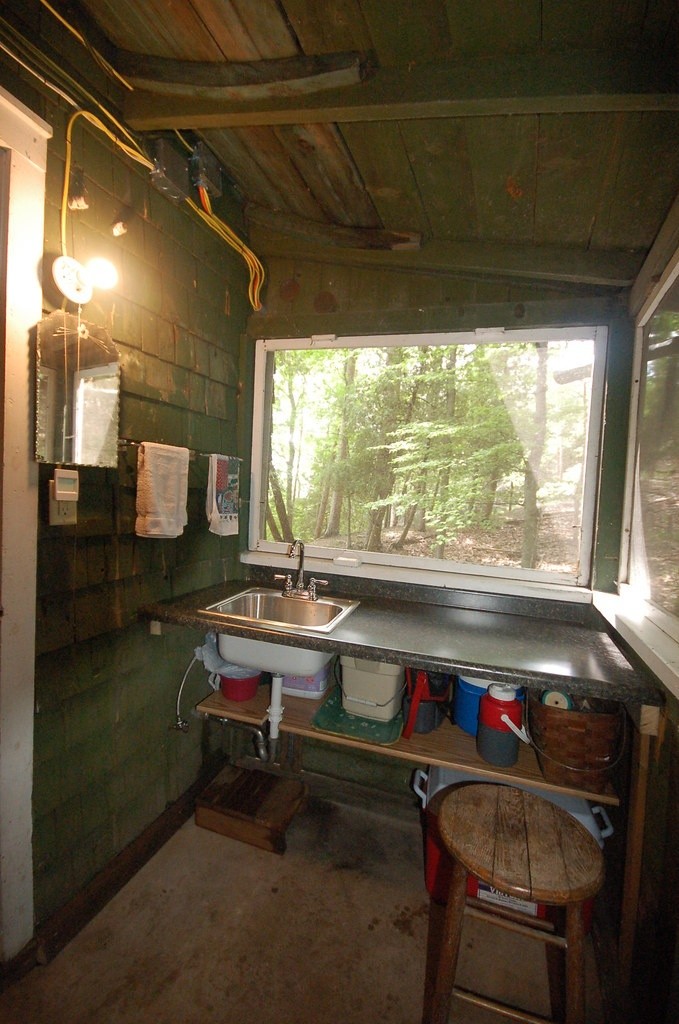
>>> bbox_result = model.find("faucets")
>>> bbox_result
[288,539,304,591]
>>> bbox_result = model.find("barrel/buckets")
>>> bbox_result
[218,673,263,702]
[525,688,627,795]
[454,676,525,736]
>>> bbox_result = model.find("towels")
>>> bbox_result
[205,452,240,537]
[134,442,188,540]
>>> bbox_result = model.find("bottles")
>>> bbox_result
[476,683,530,768]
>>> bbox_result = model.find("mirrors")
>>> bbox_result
[33,308,121,468]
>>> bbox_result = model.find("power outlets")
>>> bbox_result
[48,480,78,526]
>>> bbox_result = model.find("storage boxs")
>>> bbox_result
[413,769,614,957]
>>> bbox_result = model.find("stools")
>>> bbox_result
[428,784,607,1024]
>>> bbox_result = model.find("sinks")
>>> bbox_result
[197,587,361,679]
